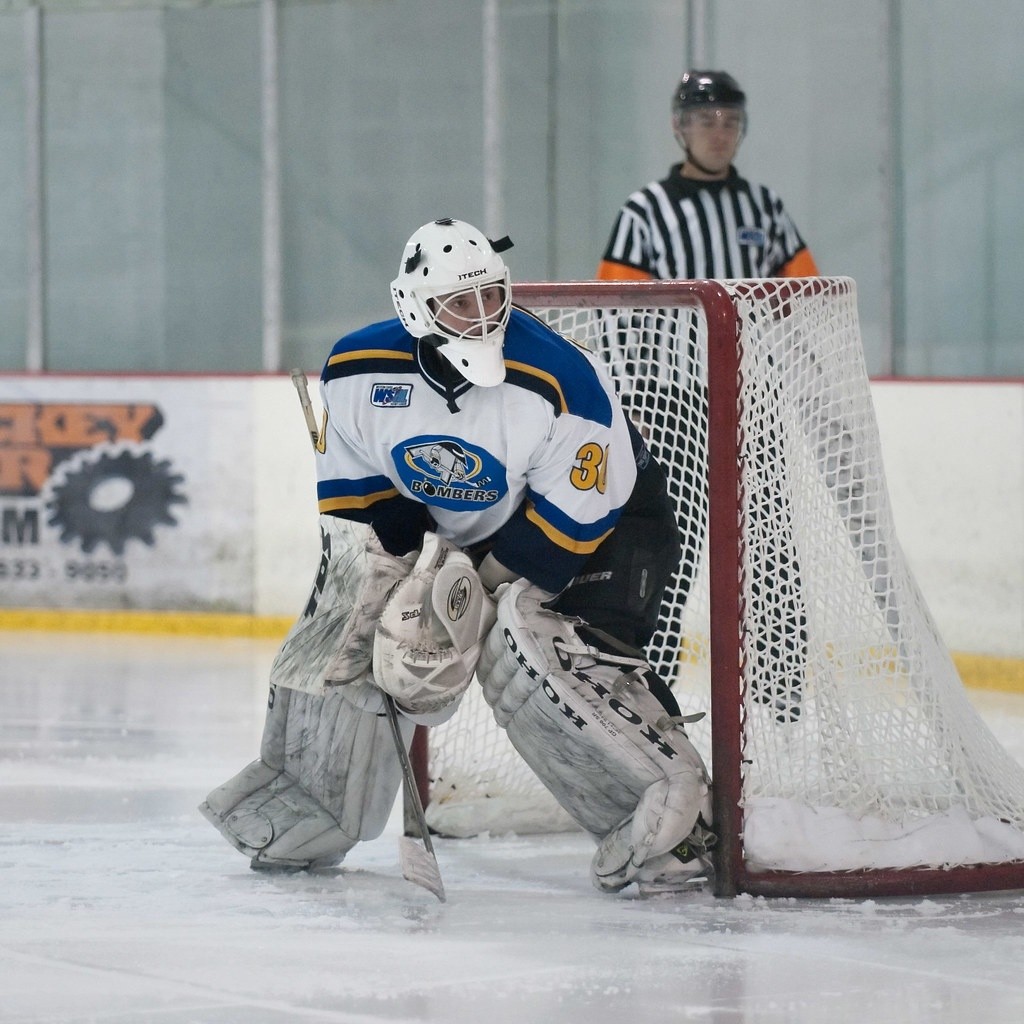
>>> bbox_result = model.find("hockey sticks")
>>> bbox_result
[291,368,448,907]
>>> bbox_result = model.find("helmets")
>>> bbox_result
[390,218,513,387]
[672,70,745,115]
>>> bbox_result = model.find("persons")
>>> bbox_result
[201,217,715,902]
[592,66,824,727]
